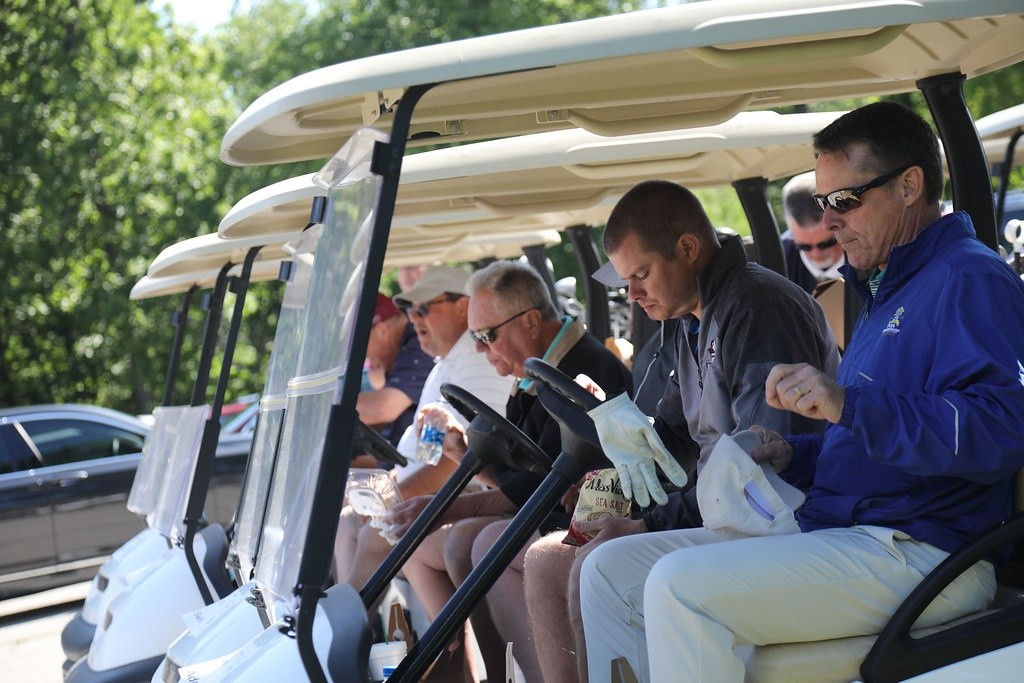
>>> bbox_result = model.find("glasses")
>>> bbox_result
[795,238,839,251]
[468,306,539,345]
[811,163,919,216]
[403,297,458,316]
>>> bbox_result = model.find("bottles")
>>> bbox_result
[416,393,451,467]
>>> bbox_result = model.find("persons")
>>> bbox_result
[577,101,1024,683]
[472,258,664,683]
[521,179,846,683]
[339,289,436,510]
[751,170,851,363]
[398,260,633,683]
[326,263,520,626]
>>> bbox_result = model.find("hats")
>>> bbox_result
[392,266,471,308]
[697,429,807,535]
[591,259,630,289]
[371,293,399,325]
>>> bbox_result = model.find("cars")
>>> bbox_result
[1,403,254,605]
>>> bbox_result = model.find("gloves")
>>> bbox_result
[587,391,688,508]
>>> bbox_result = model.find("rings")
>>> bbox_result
[794,387,804,397]
